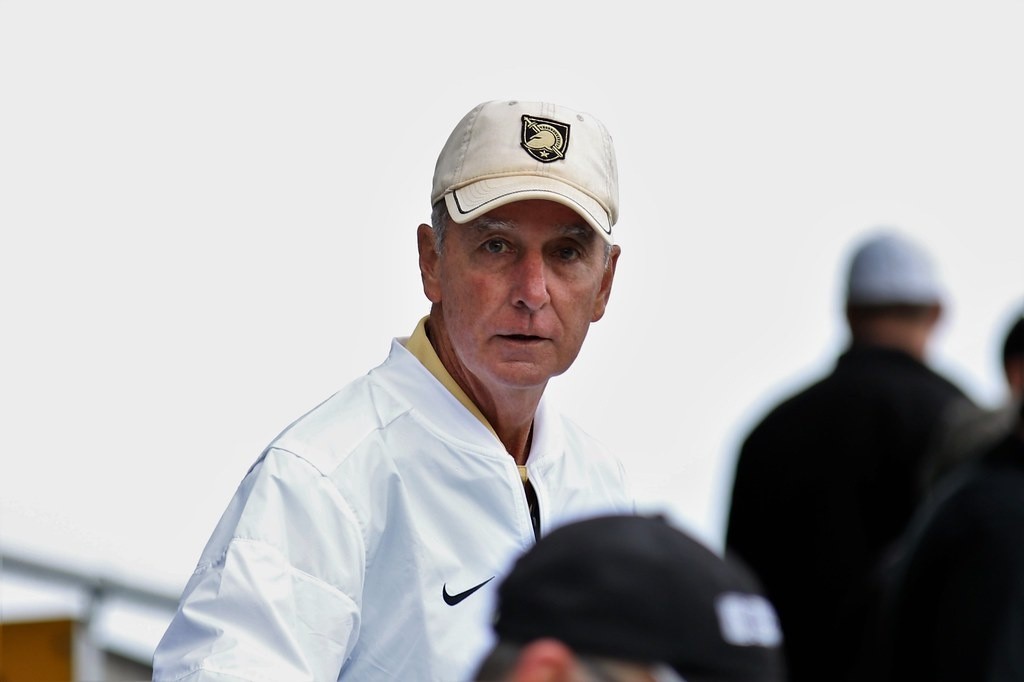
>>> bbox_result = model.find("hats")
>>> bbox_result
[491,515,784,682]
[431,100,619,246]
[849,231,947,305]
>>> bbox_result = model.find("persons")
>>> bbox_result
[470,513,769,681]
[725,234,995,680]
[152,101,659,682]
[1000,317,1024,681]
